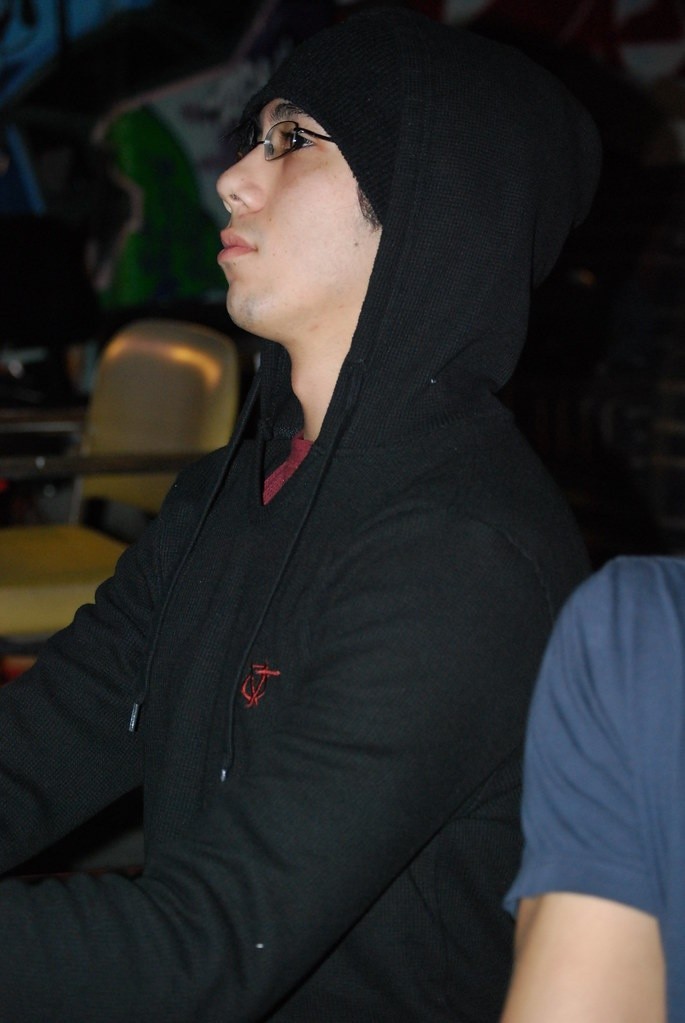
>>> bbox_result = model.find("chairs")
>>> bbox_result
[0,321,240,659]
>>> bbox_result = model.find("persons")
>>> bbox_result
[0,25,599,1023]
[500,554,685,1023]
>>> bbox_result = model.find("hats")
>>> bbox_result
[235,20,405,225]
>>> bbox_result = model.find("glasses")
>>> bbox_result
[234,117,345,161]
